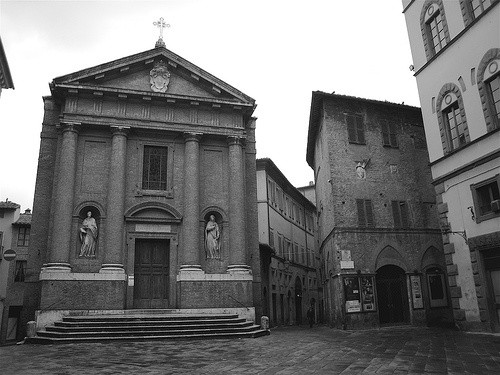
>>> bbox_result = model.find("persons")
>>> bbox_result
[307,307,313,328]
[80,210,99,257]
[204,214,221,258]
